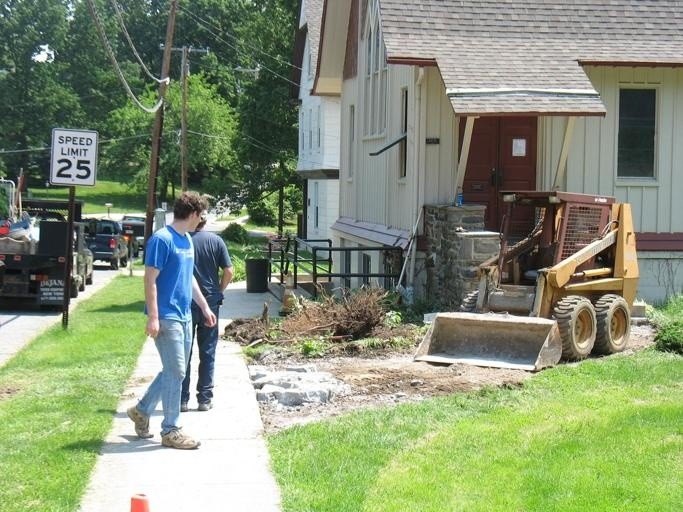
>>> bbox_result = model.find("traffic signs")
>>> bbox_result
[50,128,99,186]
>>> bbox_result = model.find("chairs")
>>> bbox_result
[524,216,589,285]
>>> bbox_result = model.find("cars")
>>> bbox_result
[119,223,139,257]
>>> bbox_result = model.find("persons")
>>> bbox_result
[126,192,218,451]
[179,208,234,413]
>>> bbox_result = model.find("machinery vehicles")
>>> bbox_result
[0,177,93,311]
[412,189,641,374]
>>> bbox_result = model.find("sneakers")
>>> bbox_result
[198,401,213,410]
[162,430,201,449]
[127,406,153,438]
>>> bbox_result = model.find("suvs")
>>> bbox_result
[119,216,146,251]
[84,217,129,270]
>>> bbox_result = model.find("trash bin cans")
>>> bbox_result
[244,258,270,293]
